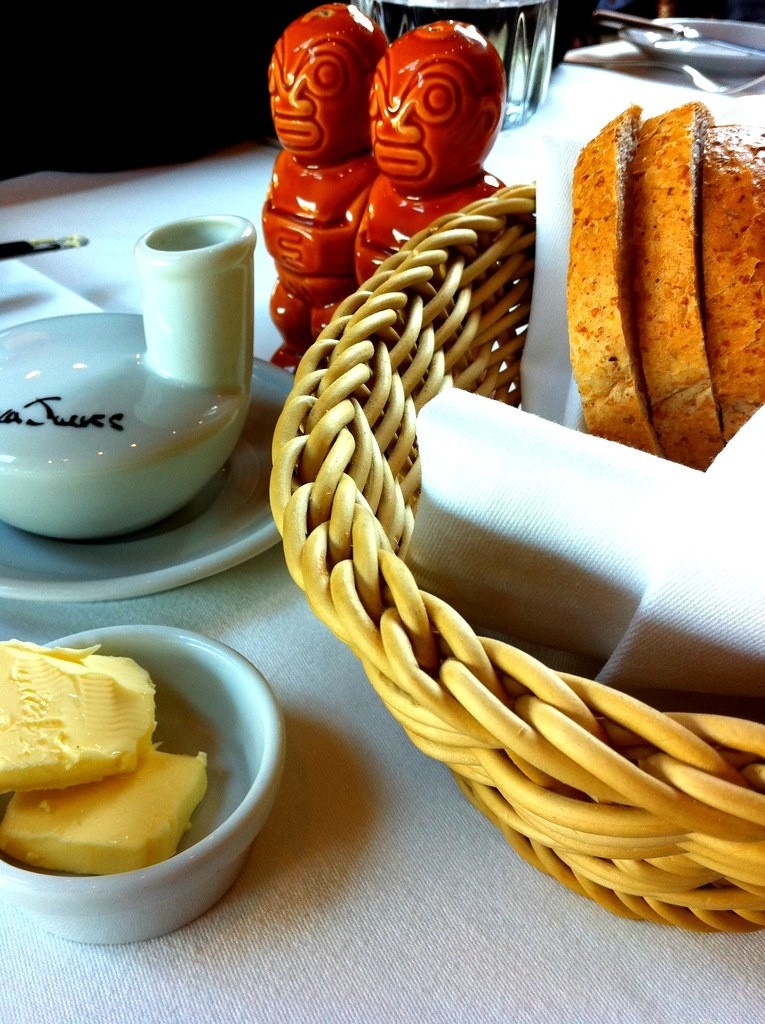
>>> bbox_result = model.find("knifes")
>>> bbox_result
[0,235,91,260]
[593,9,765,57]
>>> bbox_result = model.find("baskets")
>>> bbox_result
[268,178,765,934]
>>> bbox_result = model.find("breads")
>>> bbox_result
[568,103,765,470]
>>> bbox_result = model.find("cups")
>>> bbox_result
[342,0,556,134]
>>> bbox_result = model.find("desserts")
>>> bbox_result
[0,752,208,874]
[1,638,157,792]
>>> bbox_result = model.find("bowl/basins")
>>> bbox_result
[0,624,288,947]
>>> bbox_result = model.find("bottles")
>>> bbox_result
[0,213,257,540]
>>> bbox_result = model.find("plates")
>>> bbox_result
[0,261,104,329]
[0,357,302,604]
[620,16,765,78]
